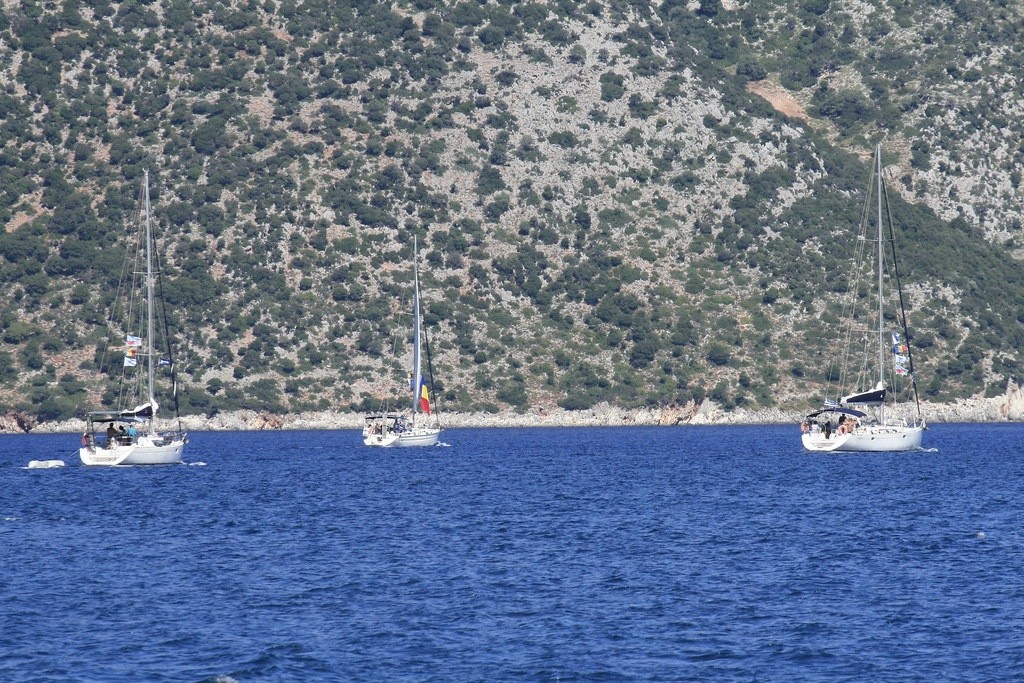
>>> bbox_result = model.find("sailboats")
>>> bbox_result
[800,138,927,454]
[361,232,445,448]
[78,166,191,466]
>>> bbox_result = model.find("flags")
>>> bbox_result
[154,356,171,368]
[122,334,143,369]
[892,331,910,377]
[420,375,431,415]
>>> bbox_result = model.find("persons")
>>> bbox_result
[394,418,405,433]
[838,413,847,424]
[822,421,831,431]
[81,432,89,448]
[366,423,383,436]
[104,421,138,448]
